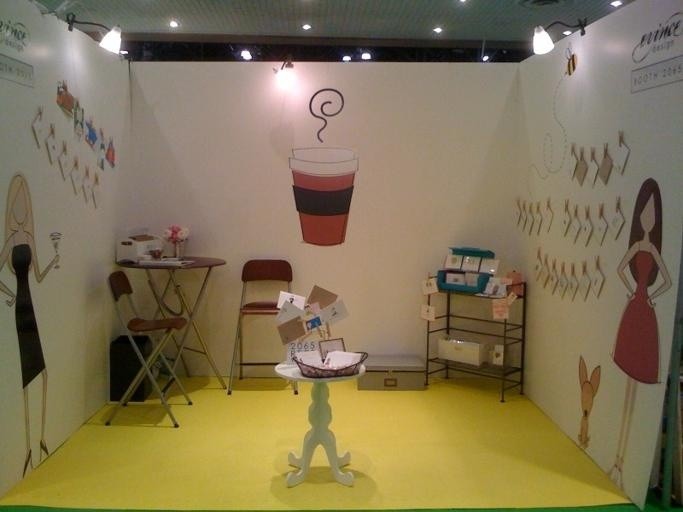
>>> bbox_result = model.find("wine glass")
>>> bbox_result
[49,232,61,267]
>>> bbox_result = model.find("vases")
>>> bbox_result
[171,240,185,259]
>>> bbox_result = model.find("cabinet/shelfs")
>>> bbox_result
[421,266,529,404]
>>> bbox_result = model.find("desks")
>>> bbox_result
[116,255,227,401]
[268,358,363,488]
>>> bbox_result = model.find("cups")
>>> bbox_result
[286,147,358,247]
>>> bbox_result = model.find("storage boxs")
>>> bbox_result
[435,332,491,368]
[436,244,495,296]
[114,234,163,262]
[356,350,427,395]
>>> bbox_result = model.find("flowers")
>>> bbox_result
[161,223,188,243]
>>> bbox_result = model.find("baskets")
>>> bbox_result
[293,353,368,377]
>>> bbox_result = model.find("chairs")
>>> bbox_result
[103,268,195,429]
[227,255,300,399]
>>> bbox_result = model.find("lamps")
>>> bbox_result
[270,51,300,91]
[529,16,586,56]
[64,10,122,57]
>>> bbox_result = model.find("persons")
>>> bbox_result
[0,175,60,478]
[604,178,671,492]
